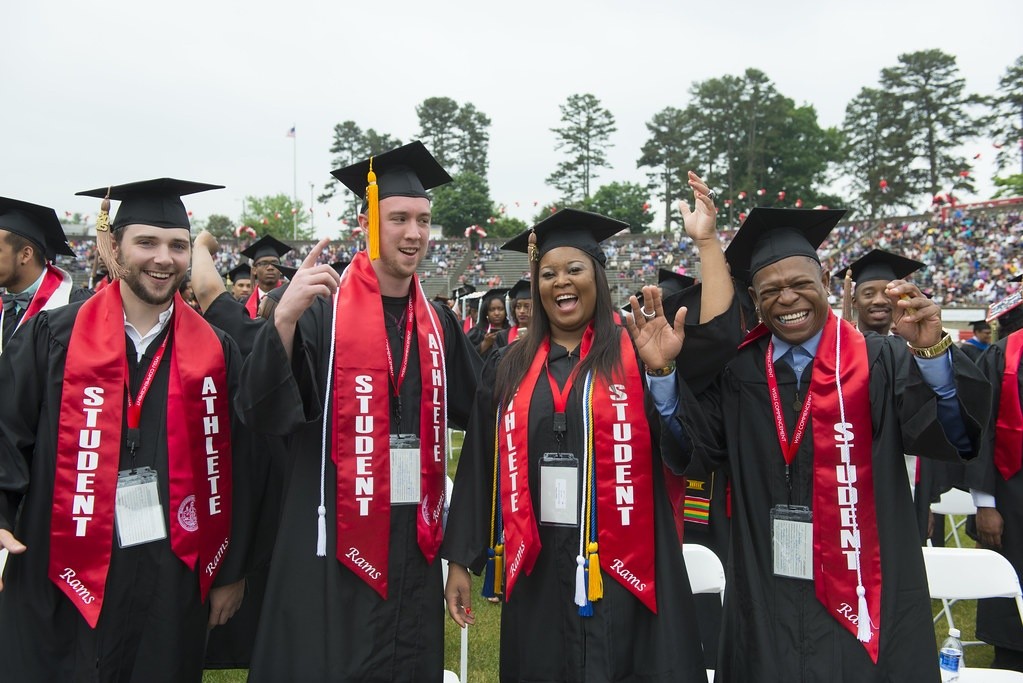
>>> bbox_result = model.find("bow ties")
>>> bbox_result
[2,293,30,312]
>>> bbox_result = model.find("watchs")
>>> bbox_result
[907,327,952,358]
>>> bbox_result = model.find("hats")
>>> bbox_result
[477,288,508,324]
[74,178,225,281]
[460,291,488,321]
[657,268,695,292]
[724,207,847,289]
[46,239,77,265]
[453,284,476,313]
[238,234,292,265]
[330,140,453,262]
[499,205,630,318]
[985,292,1023,331]
[968,320,991,332]
[221,263,258,295]
[273,262,299,282]
[505,279,533,327]
[1,195,67,257]
[836,250,926,324]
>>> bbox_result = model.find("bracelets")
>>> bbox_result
[644,360,675,376]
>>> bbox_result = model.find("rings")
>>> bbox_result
[640,306,656,318]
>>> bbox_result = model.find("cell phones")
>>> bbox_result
[517,327,527,337]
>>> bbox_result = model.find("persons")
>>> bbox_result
[0,140,1023,683]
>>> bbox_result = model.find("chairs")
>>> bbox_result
[920,484,1019,664]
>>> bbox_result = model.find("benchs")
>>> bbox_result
[416,236,534,303]
[815,204,1022,315]
[588,228,742,309]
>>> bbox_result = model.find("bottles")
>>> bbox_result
[937,627,964,683]
